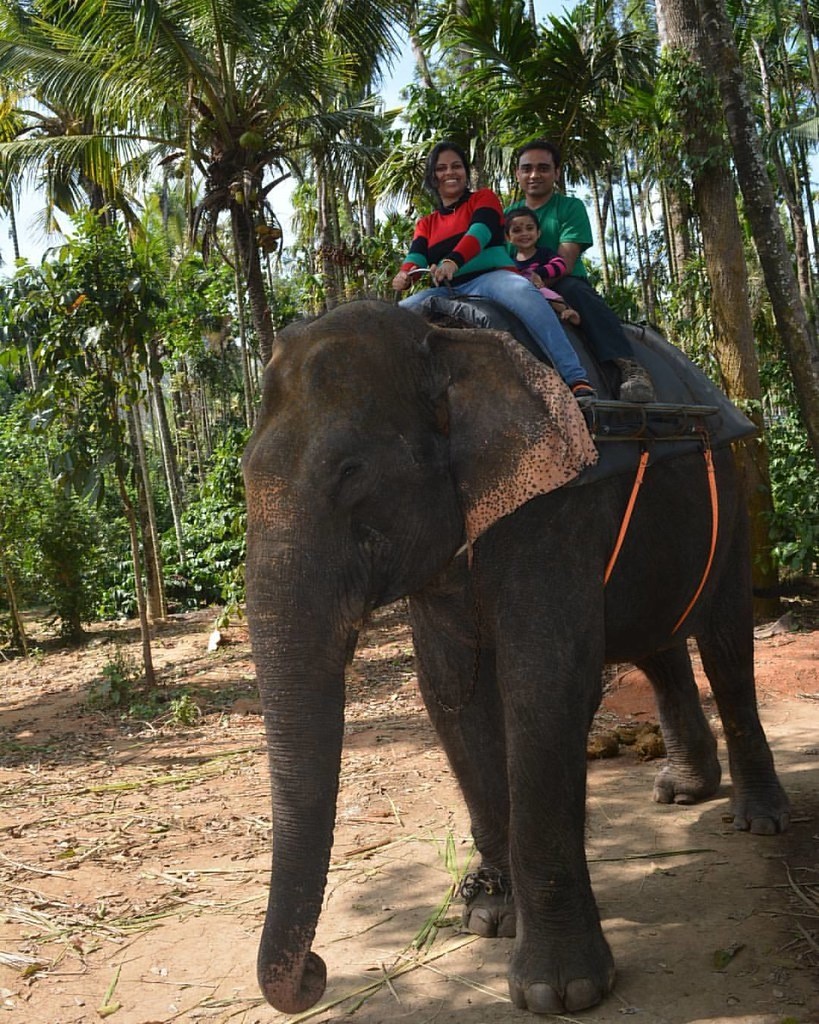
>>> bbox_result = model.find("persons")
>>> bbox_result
[504,207,581,327]
[392,142,595,396]
[503,141,657,404]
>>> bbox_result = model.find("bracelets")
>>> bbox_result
[442,259,459,270]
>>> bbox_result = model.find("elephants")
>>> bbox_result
[241,297,790,1015]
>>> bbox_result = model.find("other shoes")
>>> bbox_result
[572,381,598,402]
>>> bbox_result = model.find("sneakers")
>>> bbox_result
[617,358,656,403]
